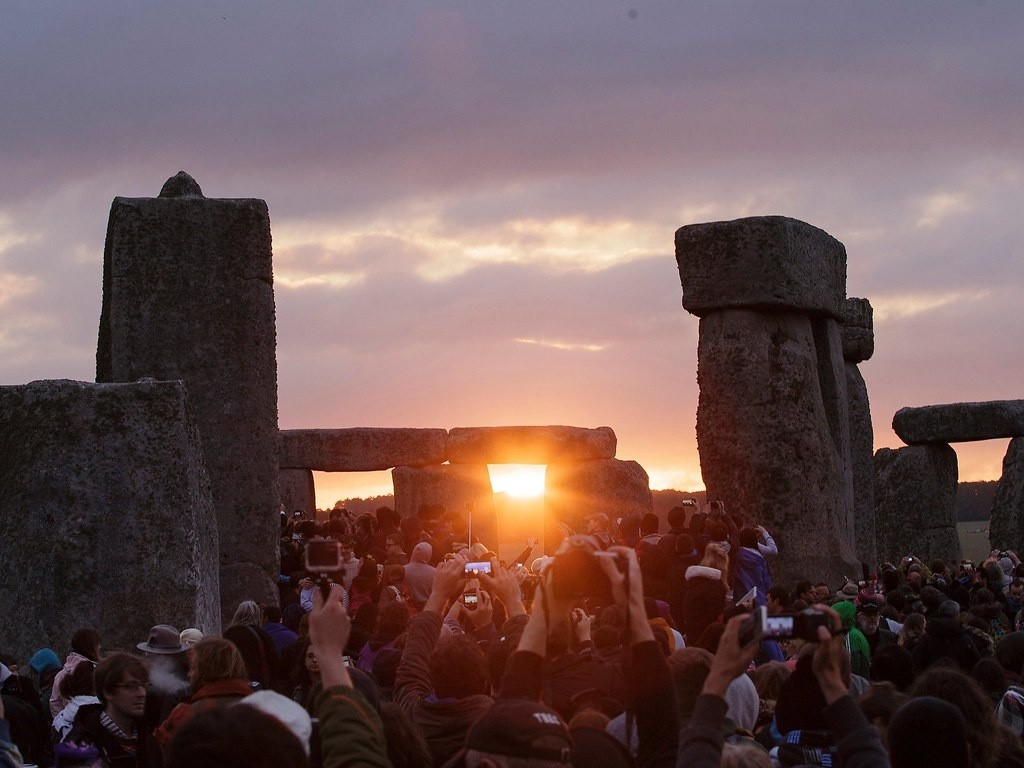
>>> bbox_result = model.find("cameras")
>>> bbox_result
[549,535,627,602]
[461,561,494,579]
[462,594,478,611]
[907,557,913,562]
[763,608,830,644]
[999,552,1008,558]
[683,500,693,506]
[517,562,523,566]
[294,510,301,516]
[961,562,974,570]
[710,501,721,509]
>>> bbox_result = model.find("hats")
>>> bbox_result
[180,628,204,646]
[836,584,858,599]
[998,557,1015,575]
[137,625,189,654]
[584,513,608,524]
[441,699,575,768]
[931,559,945,573]
[996,685,1024,735]
[854,588,879,612]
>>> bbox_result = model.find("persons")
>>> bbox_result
[0,496,1024,768]
[167,584,396,768]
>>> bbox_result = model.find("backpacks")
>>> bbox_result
[388,585,418,623]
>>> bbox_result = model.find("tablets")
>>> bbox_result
[735,586,757,606]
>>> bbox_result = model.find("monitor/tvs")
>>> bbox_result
[534,539,538,544]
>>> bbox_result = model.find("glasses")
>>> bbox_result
[385,543,396,547]
[305,654,315,661]
[340,545,350,550]
[115,682,152,690]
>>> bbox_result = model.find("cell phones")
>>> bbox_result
[738,606,768,650]
